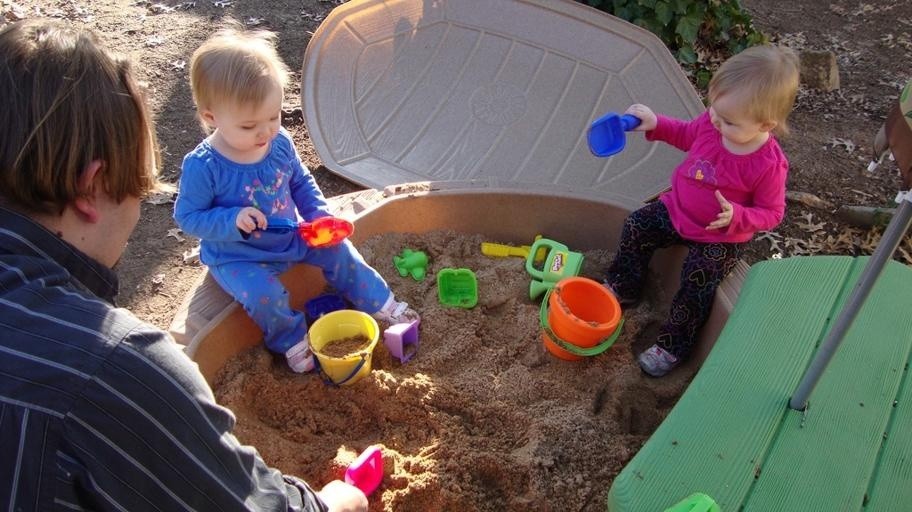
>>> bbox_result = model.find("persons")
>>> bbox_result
[1,10,372,512]
[598,43,802,379]
[170,15,423,377]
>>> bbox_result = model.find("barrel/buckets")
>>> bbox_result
[538,275,628,365]
[306,311,380,391]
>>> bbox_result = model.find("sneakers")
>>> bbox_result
[284,331,315,374]
[638,344,681,378]
[370,292,421,329]
